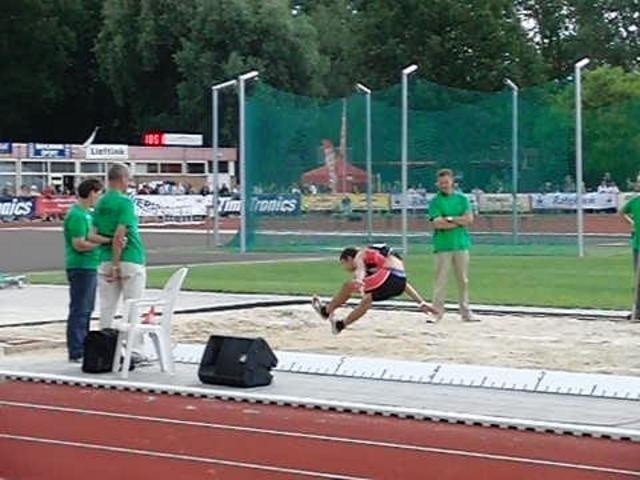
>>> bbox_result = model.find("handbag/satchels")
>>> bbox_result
[82,329,135,373]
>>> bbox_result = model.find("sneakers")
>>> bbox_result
[330,315,339,335]
[465,317,481,321]
[312,298,329,319]
[426,314,440,323]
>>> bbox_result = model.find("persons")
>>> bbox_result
[87,162,149,369]
[618,175,640,321]
[408,182,427,195]
[312,239,440,336]
[62,180,107,364]
[252,182,330,195]
[341,193,353,213]
[130,179,240,195]
[424,168,484,323]
[540,179,621,194]
[472,181,504,216]
[1,181,77,225]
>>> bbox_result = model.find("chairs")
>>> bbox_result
[110,265,188,379]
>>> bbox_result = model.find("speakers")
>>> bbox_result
[199,336,277,388]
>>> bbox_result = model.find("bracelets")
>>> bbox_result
[109,237,112,245]
[416,300,425,308]
